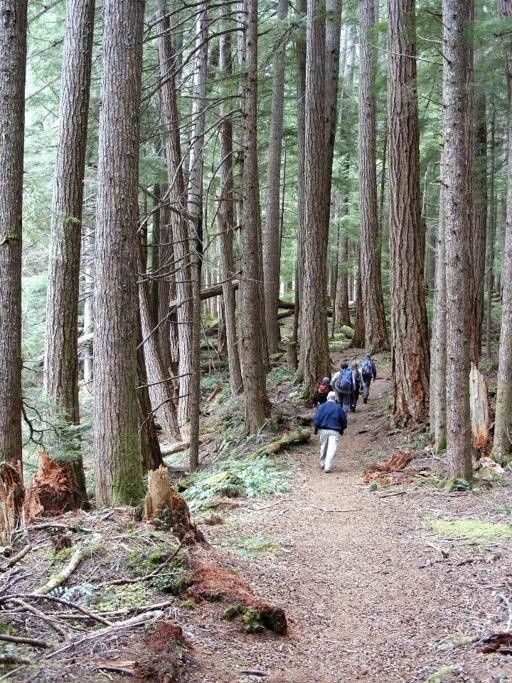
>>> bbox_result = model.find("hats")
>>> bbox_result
[323,377,330,384]
[327,391,336,401]
[340,362,348,369]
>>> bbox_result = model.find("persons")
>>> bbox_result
[315,390,348,473]
[312,354,377,409]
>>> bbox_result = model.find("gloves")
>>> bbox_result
[314,427,317,435]
